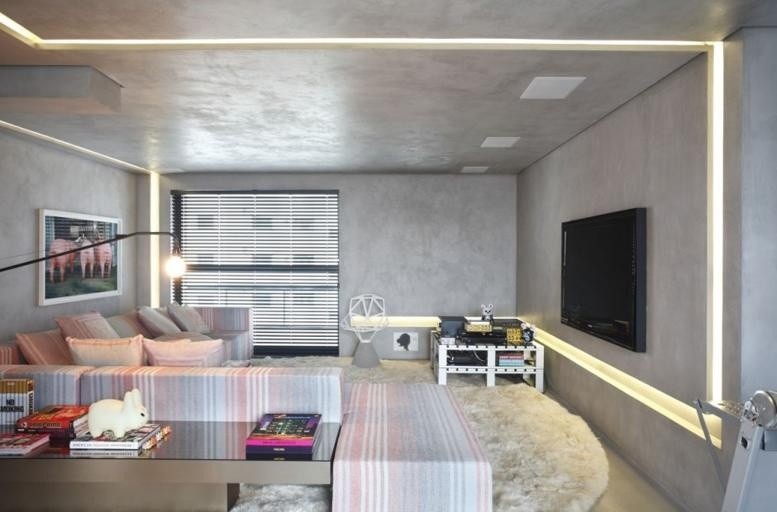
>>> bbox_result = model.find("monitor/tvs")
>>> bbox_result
[561,207,646,353]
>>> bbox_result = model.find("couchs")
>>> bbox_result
[0,303,494,512]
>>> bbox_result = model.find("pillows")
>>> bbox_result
[10,327,73,364]
[134,300,211,338]
[141,336,225,368]
[63,333,145,366]
[52,308,121,340]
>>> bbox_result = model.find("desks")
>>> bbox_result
[0,418,343,512]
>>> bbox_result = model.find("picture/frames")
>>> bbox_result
[37,208,123,307]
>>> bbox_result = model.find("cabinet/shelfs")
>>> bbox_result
[429,329,546,394]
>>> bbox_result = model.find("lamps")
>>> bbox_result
[0,230,187,280]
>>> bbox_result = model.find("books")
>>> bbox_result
[0,378,171,455]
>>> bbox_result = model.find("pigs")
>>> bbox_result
[94,235,113,280]
[48,236,82,289]
[80,233,95,279]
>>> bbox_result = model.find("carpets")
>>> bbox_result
[218,354,612,512]
